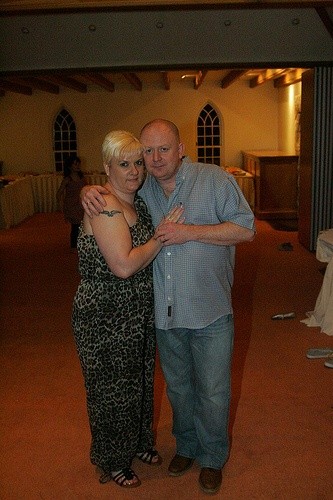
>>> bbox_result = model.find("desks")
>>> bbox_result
[0,167,255,230]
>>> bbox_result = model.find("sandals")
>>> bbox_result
[135,449,163,465]
[109,469,141,487]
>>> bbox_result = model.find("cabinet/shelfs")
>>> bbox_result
[241,150,298,219]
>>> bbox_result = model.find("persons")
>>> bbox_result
[56,153,89,251]
[78,118,257,495]
[71,128,186,488]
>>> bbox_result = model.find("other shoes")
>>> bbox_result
[307,349,333,359]
[272,312,294,319]
[281,243,293,251]
[324,360,333,367]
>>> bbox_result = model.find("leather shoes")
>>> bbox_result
[168,456,194,476]
[199,467,221,493]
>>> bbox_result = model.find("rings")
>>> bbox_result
[163,236,166,241]
